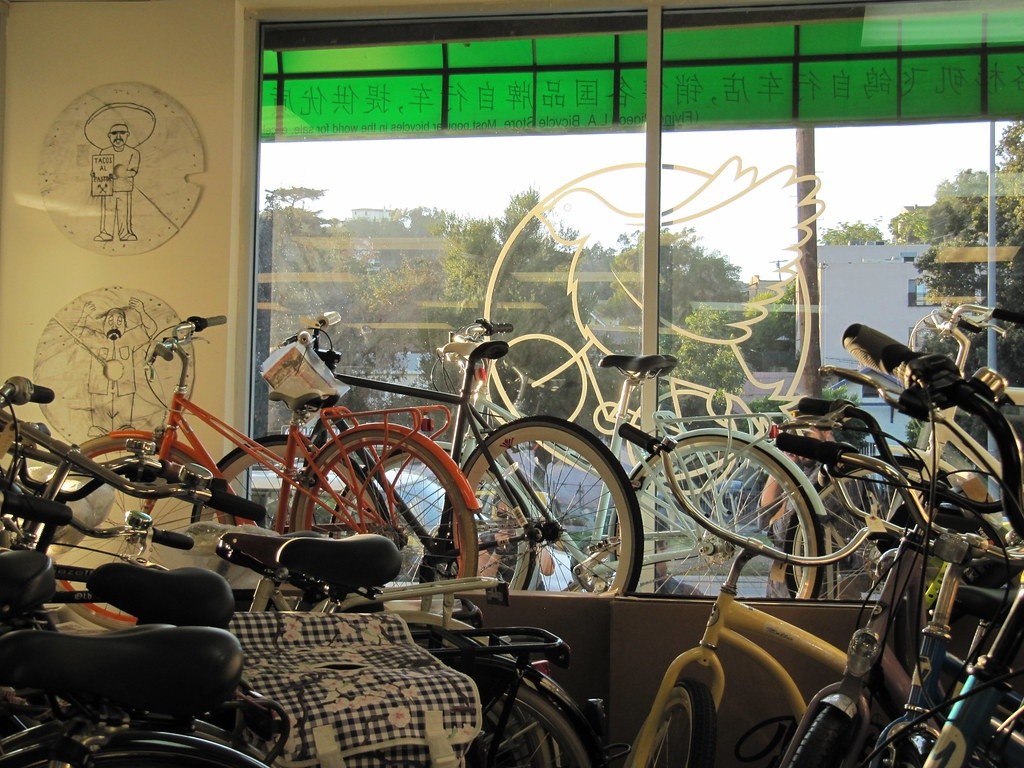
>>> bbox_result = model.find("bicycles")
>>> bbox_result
[1,287,1024,768]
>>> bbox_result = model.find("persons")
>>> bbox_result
[753,420,880,604]
[477,492,554,592]
